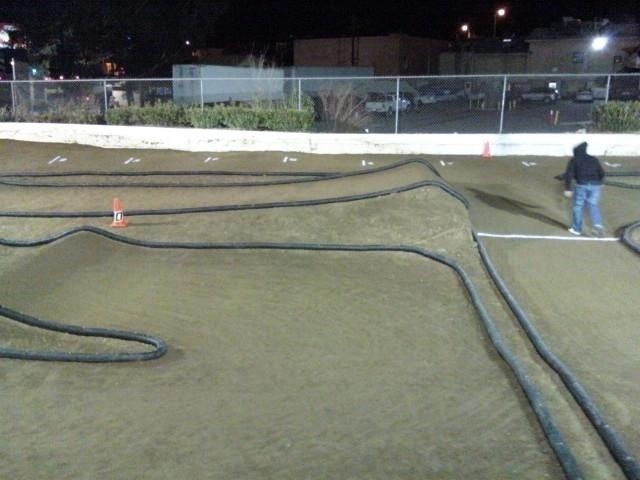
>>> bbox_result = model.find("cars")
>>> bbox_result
[312,86,487,113]
[575,88,595,102]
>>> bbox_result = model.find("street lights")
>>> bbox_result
[492,7,506,39]
[460,23,470,39]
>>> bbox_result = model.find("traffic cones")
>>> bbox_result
[482,141,491,159]
[110,196,130,227]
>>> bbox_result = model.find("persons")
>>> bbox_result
[564,142,605,236]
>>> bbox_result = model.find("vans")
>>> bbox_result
[523,89,560,105]
